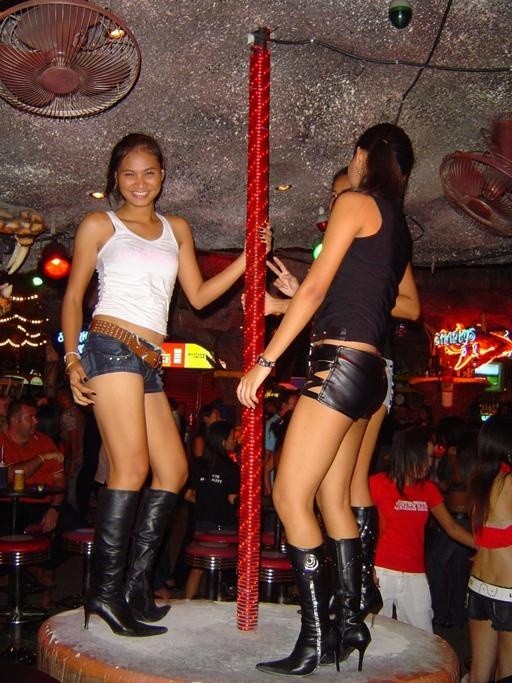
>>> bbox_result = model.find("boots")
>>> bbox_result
[83,484,179,641]
[256,504,384,678]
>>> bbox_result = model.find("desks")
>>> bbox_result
[1,483,66,619]
[237,492,284,550]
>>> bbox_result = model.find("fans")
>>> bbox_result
[437,109,511,238]
[1,0,143,122]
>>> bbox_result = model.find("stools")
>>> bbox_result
[179,529,236,601]
[259,530,297,603]
[61,526,97,605]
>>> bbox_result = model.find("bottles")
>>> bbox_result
[442,363,453,407]
[1,462,9,491]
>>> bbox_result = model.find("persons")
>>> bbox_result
[233,123,421,675]
[238,162,396,629]
[59,132,274,636]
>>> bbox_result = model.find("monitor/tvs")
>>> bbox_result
[474,362,502,392]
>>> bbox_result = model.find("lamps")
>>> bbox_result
[38,232,74,283]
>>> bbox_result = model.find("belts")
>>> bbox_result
[467,576,512,604]
[87,320,163,369]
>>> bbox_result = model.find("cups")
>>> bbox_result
[14,469,25,491]
[32,482,49,495]
